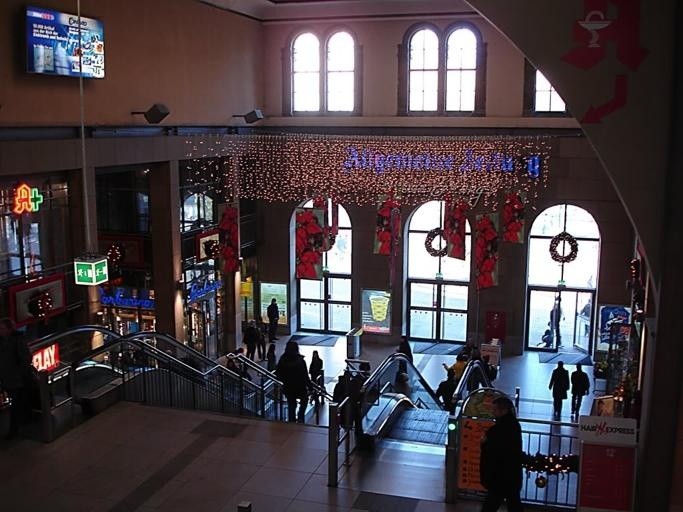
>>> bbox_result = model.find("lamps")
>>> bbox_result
[129,102,170,124]
[230,108,264,124]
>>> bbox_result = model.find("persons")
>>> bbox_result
[550,304,564,348]
[570,362,590,420]
[0,317,34,437]
[580,298,590,336]
[548,360,569,418]
[479,396,526,511]
[541,329,553,348]
[227,297,498,423]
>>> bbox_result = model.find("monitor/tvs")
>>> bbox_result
[22,5,105,78]
